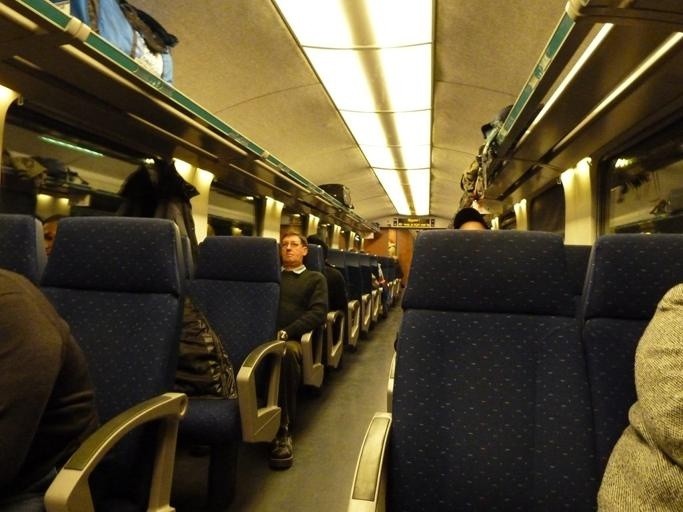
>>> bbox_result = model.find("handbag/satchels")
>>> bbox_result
[48,0,175,88]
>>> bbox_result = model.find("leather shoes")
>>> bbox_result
[270,433,294,469]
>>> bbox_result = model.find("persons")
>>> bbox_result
[307,235,347,345]
[43,214,67,258]
[0,268,99,512]
[596,284,683,512]
[350,249,388,305]
[269,233,329,470]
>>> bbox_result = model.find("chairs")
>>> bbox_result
[344,232,683,512]
[2,217,187,512]
[185,236,342,449]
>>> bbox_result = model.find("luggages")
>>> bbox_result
[318,183,355,209]
